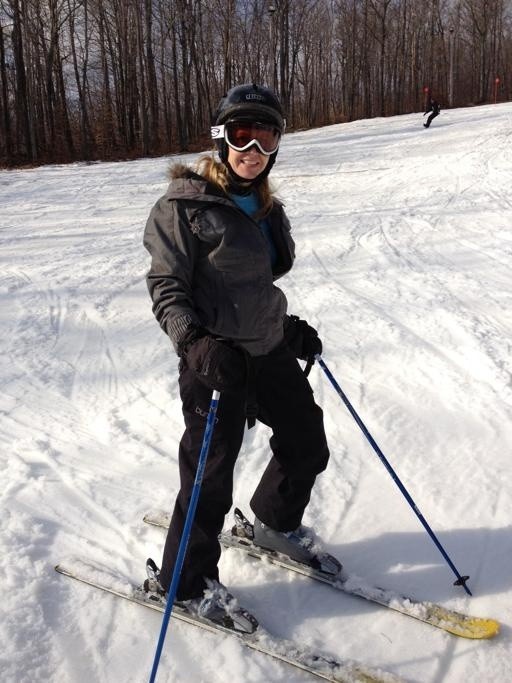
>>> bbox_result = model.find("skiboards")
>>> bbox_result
[54,508,501,682]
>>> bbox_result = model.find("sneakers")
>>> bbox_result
[251,514,344,577]
[141,558,260,635]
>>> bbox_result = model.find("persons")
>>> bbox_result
[422,96,441,127]
[140,81,343,633]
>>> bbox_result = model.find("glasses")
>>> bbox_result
[223,118,282,153]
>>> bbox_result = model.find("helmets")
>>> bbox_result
[212,83,287,189]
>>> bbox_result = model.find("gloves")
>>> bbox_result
[281,313,323,368]
[177,323,243,392]
